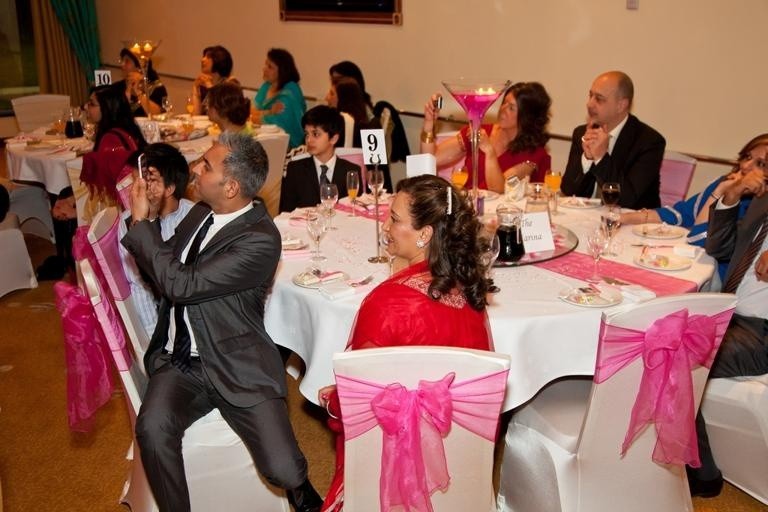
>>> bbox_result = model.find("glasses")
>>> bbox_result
[86,100,99,107]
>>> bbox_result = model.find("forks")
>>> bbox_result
[351,275,374,288]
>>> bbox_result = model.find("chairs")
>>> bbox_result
[378,107,395,192]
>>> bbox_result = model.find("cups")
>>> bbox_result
[451,167,468,189]
[525,170,563,213]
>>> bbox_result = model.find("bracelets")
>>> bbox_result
[420,126,435,145]
[710,193,719,201]
[640,208,648,223]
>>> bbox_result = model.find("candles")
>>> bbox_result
[128,42,152,54]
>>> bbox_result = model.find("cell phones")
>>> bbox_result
[138,153,148,179]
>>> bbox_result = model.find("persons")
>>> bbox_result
[192,47,240,116]
[116,141,196,344]
[108,39,168,116]
[558,70,667,212]
[202,81,256,137]
[319,172,502,512]
[419,80,552,196]
[116,128,324,511]
[48,84,148,283]
[280,61,372,214]
[613,131,768,294]
[684,143,768,500]
[250,48,307,152]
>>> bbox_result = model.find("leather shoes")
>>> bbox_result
[288,483,324,511]
[689,468,722,499]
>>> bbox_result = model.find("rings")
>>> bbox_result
[581,135,585,142]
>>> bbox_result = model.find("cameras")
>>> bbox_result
[433,96,442,109]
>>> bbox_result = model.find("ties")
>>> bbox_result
[724,219,767,295]
[320,165,330,194]
[170,213,216,372]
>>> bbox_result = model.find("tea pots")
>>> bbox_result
[493,203,525,265]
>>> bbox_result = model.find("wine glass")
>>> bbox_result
[439,79,511,218]
[123,39,162,123]
[274,171,383,262]
[8,94,263,155]
[479,234,500,279]
[588,183,622,284]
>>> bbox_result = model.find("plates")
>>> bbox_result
[632,224,688,240]
[292,274,349,290]
[556,196,600,208]
[461,190,499,200]
[634,252,692,271]
[559,285,623,308]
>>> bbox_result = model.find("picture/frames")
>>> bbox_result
[279,0,403,26]
